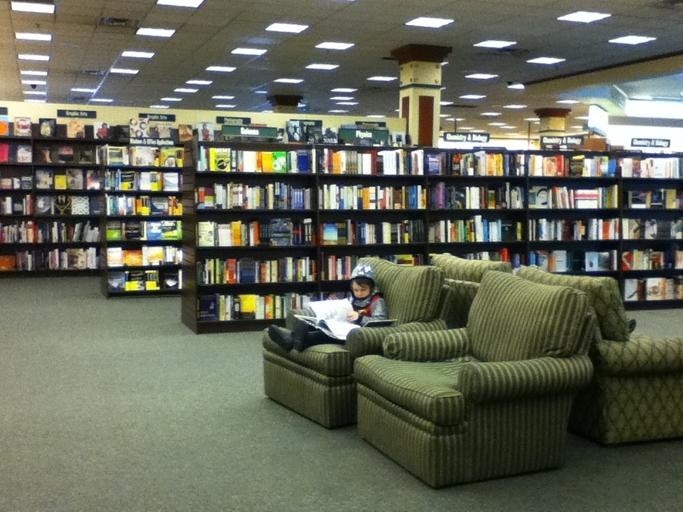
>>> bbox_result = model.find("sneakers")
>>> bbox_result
[269,325,294,350]
[294,320,307,352]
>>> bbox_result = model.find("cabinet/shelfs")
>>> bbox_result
[0,116,683,333]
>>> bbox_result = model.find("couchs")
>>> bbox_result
[519,258,683,446]
[426,249,515,308]
[263,255,456,435]
[354,268,597,488]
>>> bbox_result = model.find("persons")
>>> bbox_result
[267,262,388,352]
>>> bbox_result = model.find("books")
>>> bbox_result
[428,152,525,268]
[1,116,110,270]
[528,153,619,273]
[105,145,185,289]
[620,157,683,301]
[317,148,427,299]
[200,144,315,320]
[294,298,362,340]
[130,117,213,145]
